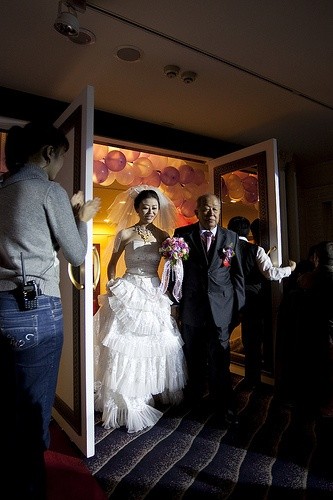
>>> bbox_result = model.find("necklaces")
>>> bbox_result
[135,223,154,241]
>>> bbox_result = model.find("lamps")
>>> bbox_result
[53,0,86,37]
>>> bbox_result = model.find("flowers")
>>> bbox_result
[159,237,189,303]
[223,242,235,268]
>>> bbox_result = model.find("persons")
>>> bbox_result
[0,120,95,500]
[251,217,272,363]
[93,185,187,433]
[229,216,333,419]
[166,193,246,400]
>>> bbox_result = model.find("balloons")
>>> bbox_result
[93,160,108,183]
[116,164,134,185]
[219,170,259,212]
[121,149,140,162]
[93,144,108,160]
[98,161,117,186]
[131,143,209,217]
[105,150,126,172]
